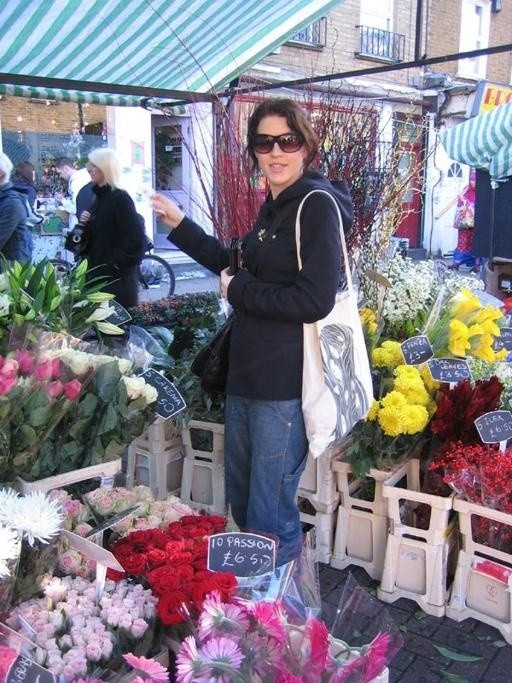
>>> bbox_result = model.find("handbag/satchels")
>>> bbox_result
[295,187,374,459]
[193,239,240,396]
[64,206,94,255]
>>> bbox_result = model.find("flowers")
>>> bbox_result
[44,348,159,466]
[0,251,125,347]
[215,238,510,552]
[0,485,390,683]
[0,348,82,484]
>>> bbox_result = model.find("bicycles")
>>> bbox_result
[32,240,178,308]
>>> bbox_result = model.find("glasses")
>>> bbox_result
[252,132,305,154]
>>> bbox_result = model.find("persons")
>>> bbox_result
[55,155,91,264]
[447,172,483,272]
[12,161,52,258]
[150,99,354,622]
[0,151,38,276]
[80,146,144,308]
